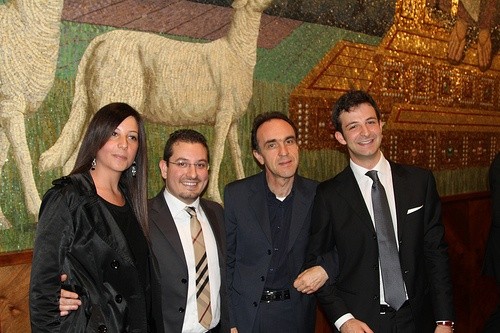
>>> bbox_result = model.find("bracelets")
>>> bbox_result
[437,321,455,326]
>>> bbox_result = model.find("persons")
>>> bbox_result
[303,90,456,333]
[222,110,339,333]
[59,128,227,333]
[28,102,150,333]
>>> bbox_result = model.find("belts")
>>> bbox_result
[260,290,291,301]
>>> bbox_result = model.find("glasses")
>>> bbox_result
[169,160,210,170]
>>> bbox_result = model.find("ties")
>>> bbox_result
[364,170,406,310]
[184,206,213,329]
